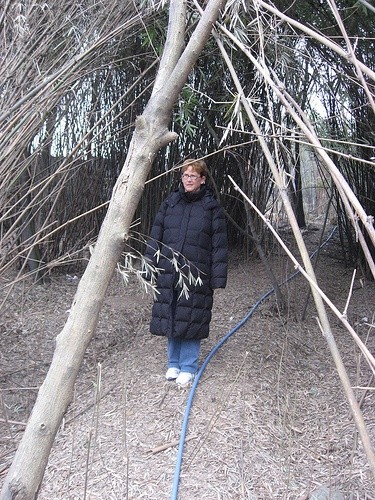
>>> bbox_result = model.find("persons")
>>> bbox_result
[139,159,228,384]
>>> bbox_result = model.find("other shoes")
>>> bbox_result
[176,372,194,387]
[166,368,180,380]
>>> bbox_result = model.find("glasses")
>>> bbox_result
[182,174,201,180]
